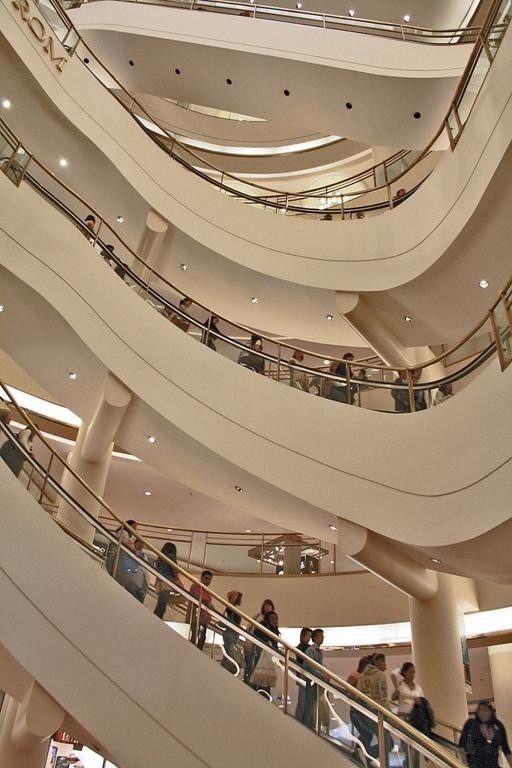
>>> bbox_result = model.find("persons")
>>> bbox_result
[184,570,214,650]
[80,215,96,239]
[237,333,265,374]
[391,370,408,413]
[0,422,41,476]
[458,699,512,768]
[154,542,179,619]
[176,298,193,312]
[199,315,225,350]
[391,660,426,767]
[99,244,115,267]
[356,211,366,219]
[115,520,149,604]
[435,382,455,406]
[284,345,369,407]
[354,653,396,768]
[243,599,281,697]
[411,367,428,412]
[392,188,408,207]
[0,399,16,426]
[114,263,132,286]
[346,655,374,740]
[319,214,333,220]
[293,627,331,731]
[220,591,243,676]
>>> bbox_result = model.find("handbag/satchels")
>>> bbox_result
[250,649,277,687]
